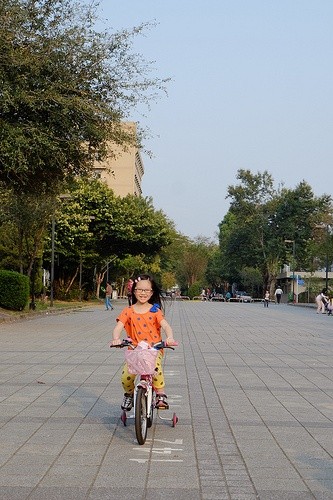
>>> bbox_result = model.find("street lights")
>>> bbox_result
[284,239,295,304]
[315,223,329,297]
[78,216,96,304]
[50,193,72,308]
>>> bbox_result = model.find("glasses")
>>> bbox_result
[134,289,152,292]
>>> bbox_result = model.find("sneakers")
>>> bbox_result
[121,396,133,411]
[156,394,168,407]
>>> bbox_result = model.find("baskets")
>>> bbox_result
[125,350,160,375]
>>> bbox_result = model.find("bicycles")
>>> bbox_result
[108,337,179,445]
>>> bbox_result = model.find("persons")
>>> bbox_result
[274,286,283,304]
[103,281,114,311]
[263,289,270,308]
[112,273,175,411]
[200,287,231,303]
[126,277,134,306]
[314,287,333,316]
